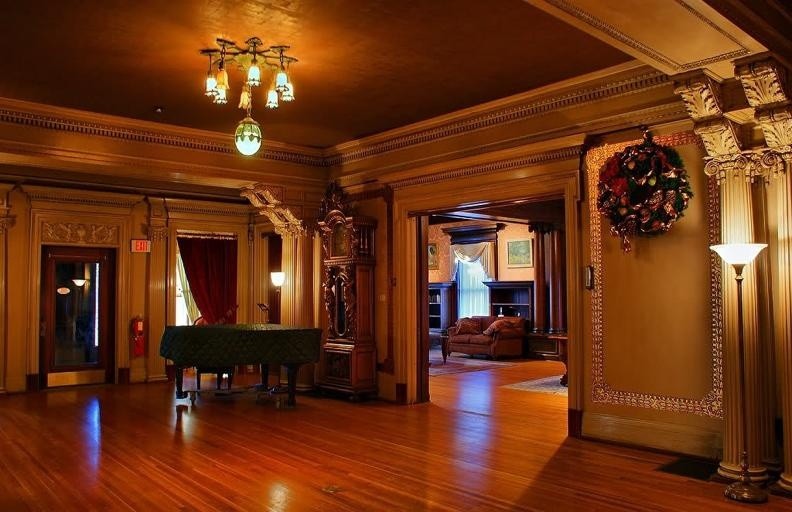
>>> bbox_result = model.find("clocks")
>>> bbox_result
[315,183,375,264]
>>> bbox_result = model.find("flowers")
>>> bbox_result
[594,143,689,238]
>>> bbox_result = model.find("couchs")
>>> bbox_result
[436,316,525,366]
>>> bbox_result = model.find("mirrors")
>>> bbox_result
[41,247,114,391]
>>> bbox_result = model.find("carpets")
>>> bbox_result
[499,375,569,395]
[428,345,519,378]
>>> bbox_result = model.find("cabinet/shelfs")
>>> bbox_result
[483,280,534,330]
[427,282,456,331]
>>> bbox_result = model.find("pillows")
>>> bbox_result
[456,317,515,335]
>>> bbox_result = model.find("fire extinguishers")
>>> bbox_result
[128,315,145,357]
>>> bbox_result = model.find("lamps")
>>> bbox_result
[197,37,299,155]
[73,278,87,343]
[270,272,289,393]
[709,239,770,503]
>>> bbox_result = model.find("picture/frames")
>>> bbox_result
[427,242,440,270]
[506,238,534,268]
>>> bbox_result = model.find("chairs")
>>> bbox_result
[194,316,235,394]
